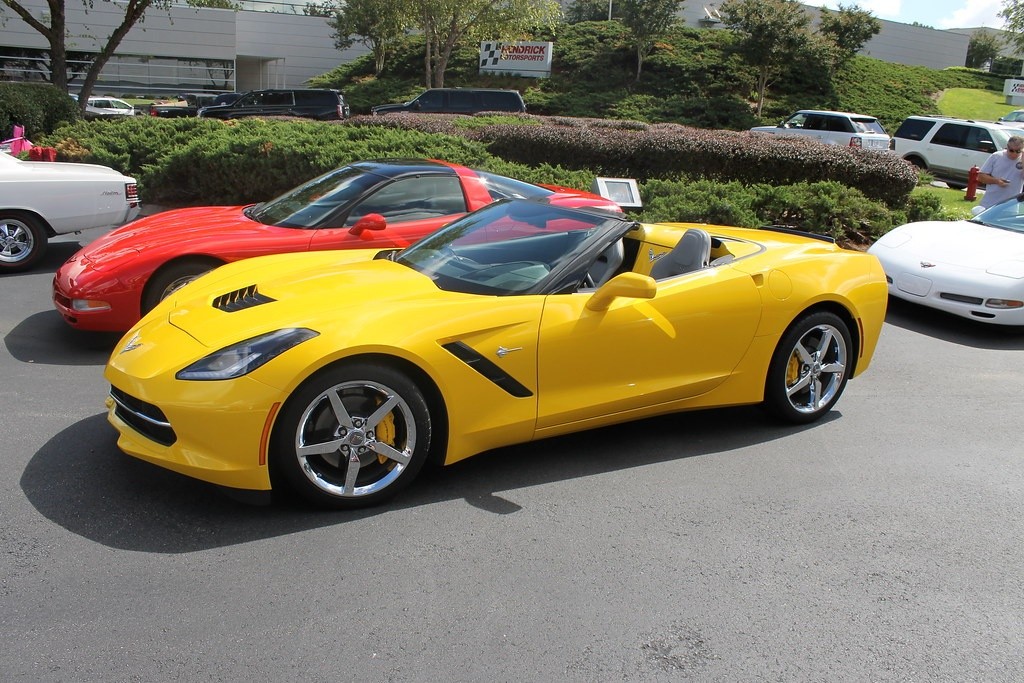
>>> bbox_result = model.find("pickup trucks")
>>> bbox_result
[150,93,218,119]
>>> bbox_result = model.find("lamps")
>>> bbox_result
[592,177,643,214]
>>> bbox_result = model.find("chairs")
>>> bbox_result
[547,229,626,291]
[649,228,710,283]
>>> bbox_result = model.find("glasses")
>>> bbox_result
[1008,145,1020,153]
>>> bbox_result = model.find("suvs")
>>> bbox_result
[371,88,527,117]
[751,111,890,152]
[200,90,349,122]
[888,116,1024,191]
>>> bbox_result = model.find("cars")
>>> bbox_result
[997,109,1024,130]
[0,143,142,270]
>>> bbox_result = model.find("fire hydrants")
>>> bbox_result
[963,165,981,203]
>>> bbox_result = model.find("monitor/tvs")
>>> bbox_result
[605,181,633,203]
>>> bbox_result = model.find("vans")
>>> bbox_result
[68,94,141,116]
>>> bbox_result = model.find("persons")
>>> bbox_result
[976,135,1024,209]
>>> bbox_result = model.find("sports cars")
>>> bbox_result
[104,197,888,508]
[51,158,624,334]
[867,191,1024,327]
